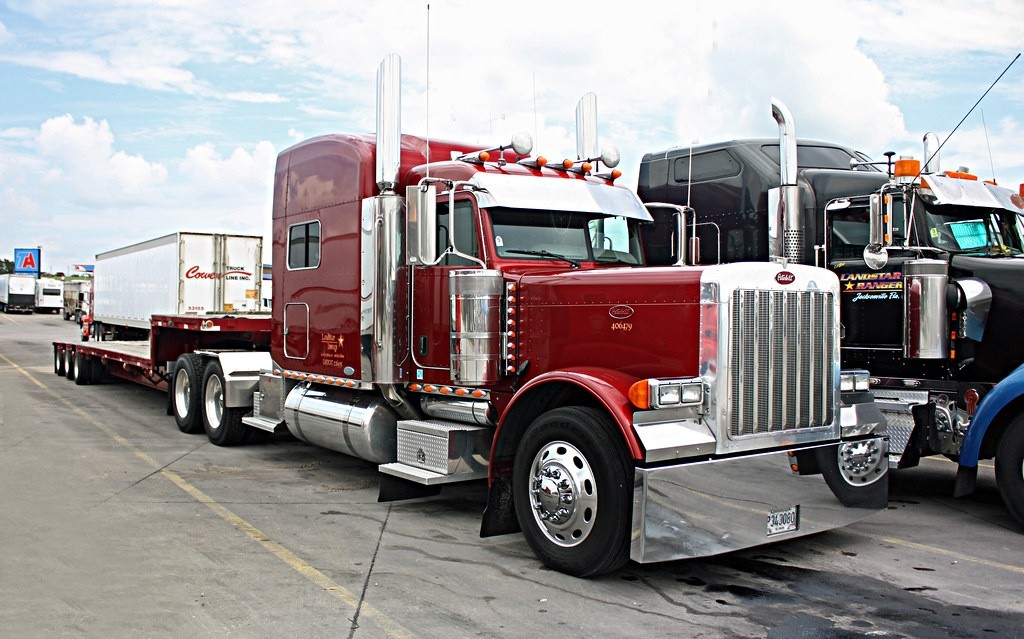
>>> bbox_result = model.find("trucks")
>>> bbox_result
[0,274,36,314]
[63,280,91,324]
[79,231,264,342]
[35,278,64,314]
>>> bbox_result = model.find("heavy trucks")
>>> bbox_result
[627,95,1024,534]
[52,52,889,583]
